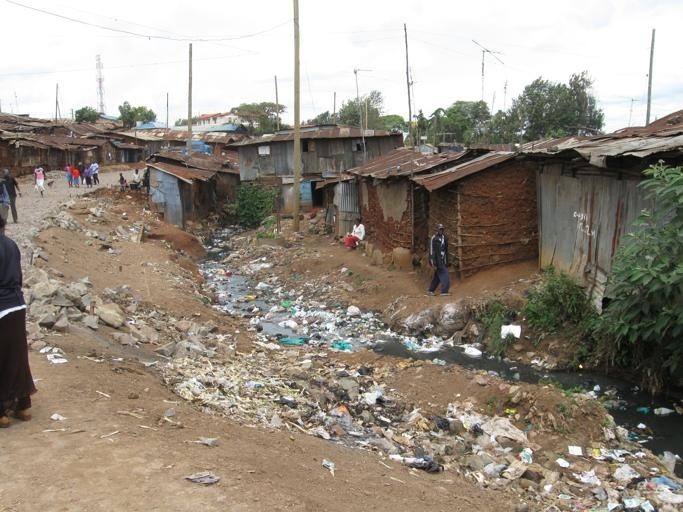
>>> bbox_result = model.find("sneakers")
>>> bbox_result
[441,292,451,296]
[427,290,436,296]
[0,415,10,428]
[8,408,32,420]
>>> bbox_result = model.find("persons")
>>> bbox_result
[1,203,40,429]
[0,177,11,237]
[117,172,128,192]
[426,223,451,297]
[63,159,101,190]
[32,165,49,199]
[1,168,23,223]
[131,168,142,189]
[343,217,366,250]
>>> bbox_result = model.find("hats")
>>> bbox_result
[437,223,445,229]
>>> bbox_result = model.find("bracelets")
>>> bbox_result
[17,192,22,194]
